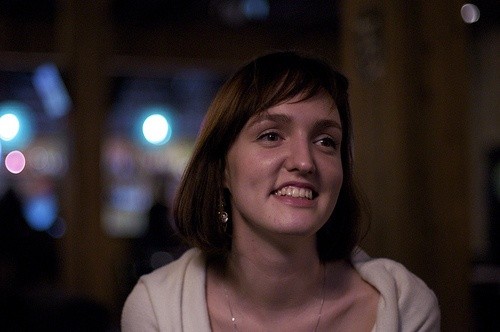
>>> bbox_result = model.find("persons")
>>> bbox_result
[120,49,443,332]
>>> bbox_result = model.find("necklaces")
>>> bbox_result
[221,257,327,332]
